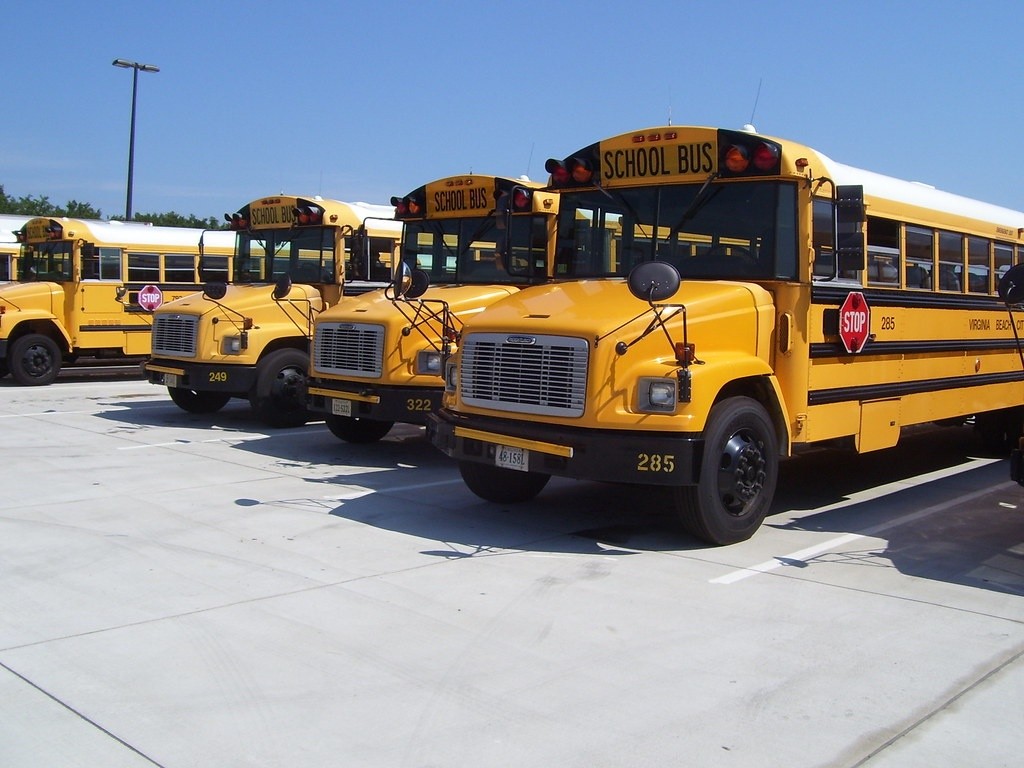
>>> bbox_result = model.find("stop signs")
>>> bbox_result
[839,291,870,353]
[138,285,163,312]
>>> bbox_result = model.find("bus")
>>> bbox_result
[383,69,1024,548]
[114,191,588,427]
[270,166,915,443]
[0,217,257,386]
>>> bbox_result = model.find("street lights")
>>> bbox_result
[113,58,160,220]
